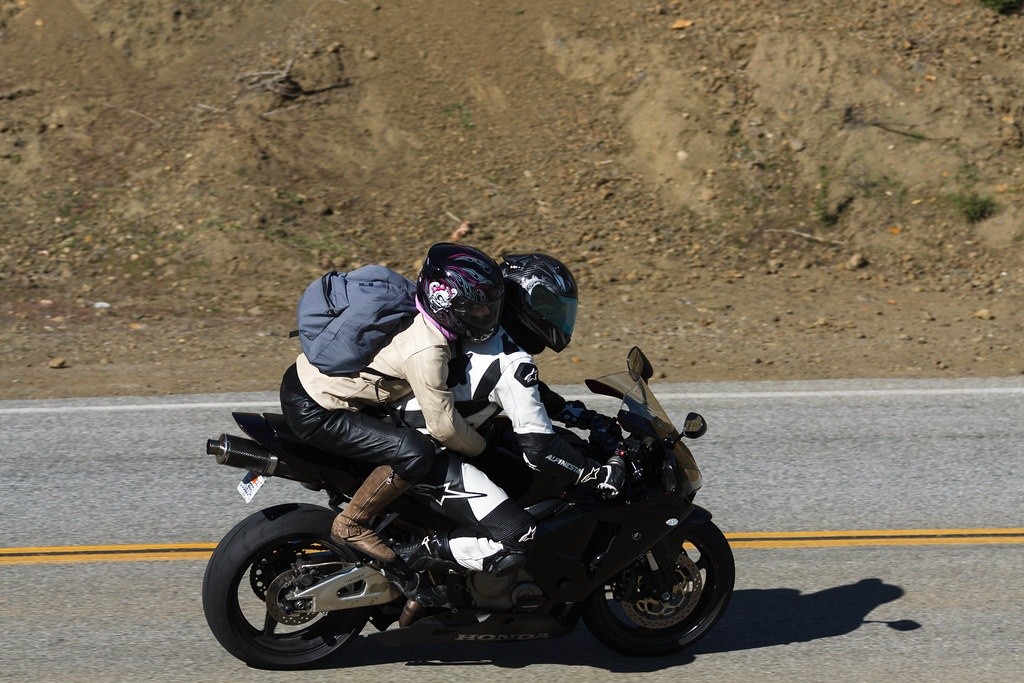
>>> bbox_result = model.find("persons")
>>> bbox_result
[363,253,625,609]
[280,242,503,562]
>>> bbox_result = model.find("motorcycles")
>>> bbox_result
[201,346,736,672]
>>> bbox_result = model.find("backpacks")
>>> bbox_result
[297,265,417,380]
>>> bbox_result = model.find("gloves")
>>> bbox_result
[575,461,626,498]
[586,410,621,432]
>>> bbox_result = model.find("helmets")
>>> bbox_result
[500,253,578,353]
[417,242,503,343]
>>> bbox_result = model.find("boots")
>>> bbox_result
[381,528,455,608]
[329,465,413,562]
